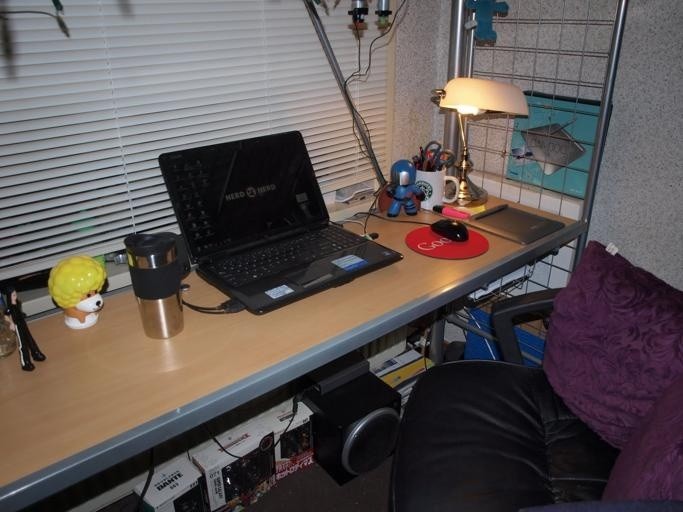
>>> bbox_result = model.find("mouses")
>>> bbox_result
[432,219,469,242]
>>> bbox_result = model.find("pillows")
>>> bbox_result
[600,377,683,504]
[542,241,683,450]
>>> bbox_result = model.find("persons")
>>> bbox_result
[3,287,46,371]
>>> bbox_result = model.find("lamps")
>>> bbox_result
[431,77,530,207]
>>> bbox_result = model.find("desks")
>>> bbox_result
[0,194,587,512]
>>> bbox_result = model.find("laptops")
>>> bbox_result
[158,131,404,315]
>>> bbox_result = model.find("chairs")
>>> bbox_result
[388,287,683,512]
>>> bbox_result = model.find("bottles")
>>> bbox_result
[123,232,185,339]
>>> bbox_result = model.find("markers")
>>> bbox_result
[432,205,469,219]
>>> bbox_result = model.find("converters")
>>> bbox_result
[301,351,369,396]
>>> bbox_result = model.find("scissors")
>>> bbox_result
[422,141,454,172]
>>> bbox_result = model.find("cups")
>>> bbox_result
[416,166,459,211]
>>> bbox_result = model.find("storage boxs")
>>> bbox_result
[371,349,435,408]
[132,400,315,512]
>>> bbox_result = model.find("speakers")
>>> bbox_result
[157,232,190,290]
[286,371,401,486]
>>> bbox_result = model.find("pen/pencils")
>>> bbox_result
[412,146,448,172]
